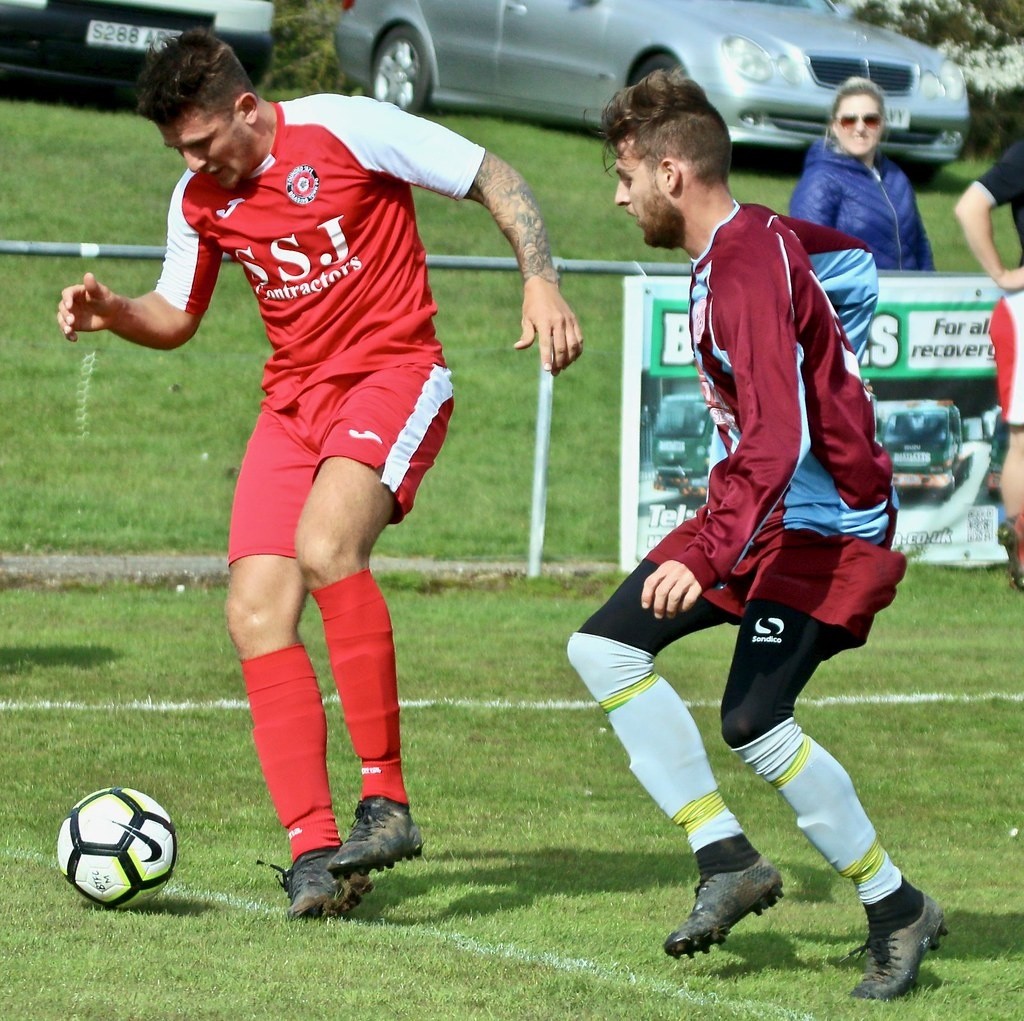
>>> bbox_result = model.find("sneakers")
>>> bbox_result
[256,847,373,919]
[327,795,423,880]
[664,853,785,960]
[998,518,1024,591]
[840,891,949,1000]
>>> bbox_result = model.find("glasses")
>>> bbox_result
[836,115,884,127]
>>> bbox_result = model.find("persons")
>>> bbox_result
[955,132,1024,594]
[566,69,950,1002]
[788,77,937,271]
[57,28,583,918]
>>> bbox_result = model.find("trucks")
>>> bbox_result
[653,393,714,488]
[882,399,974,503]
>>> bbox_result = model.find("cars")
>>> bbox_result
[0,1,275,111]
[333,0,971,189]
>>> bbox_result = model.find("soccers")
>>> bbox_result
[57,785,179,909]
